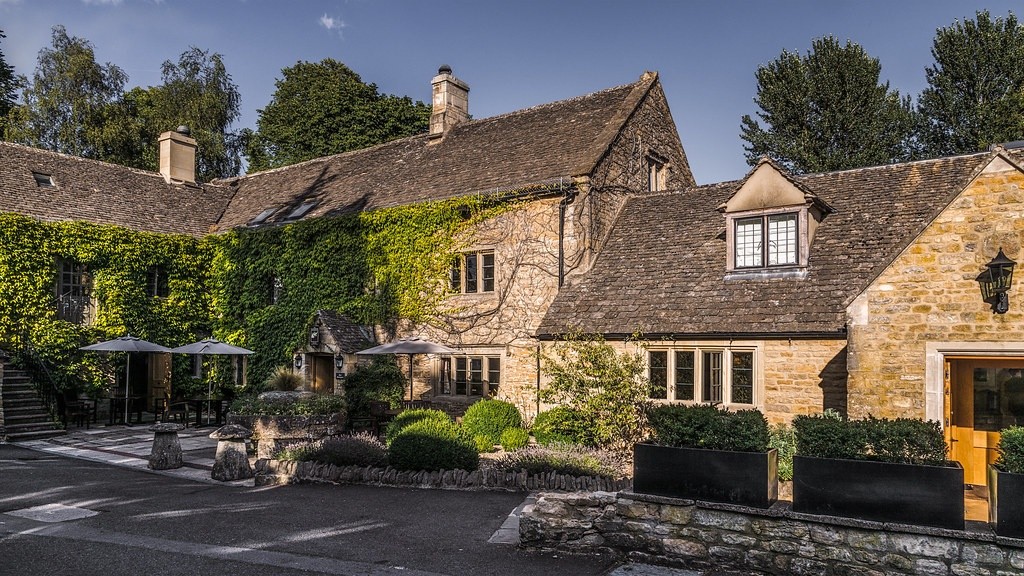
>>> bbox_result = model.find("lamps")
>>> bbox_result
[985,247,1017,314]
[334,352,344,370]
[295,354,302,369]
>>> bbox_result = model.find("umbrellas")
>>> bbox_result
[79,334,255,424]
[355,338,466,400]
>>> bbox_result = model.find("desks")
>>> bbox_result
[97,394,142,427]
[184,396,233,428]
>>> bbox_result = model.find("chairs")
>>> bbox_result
[57,390,90,430]
[113,386,134,423]
[155,386,177,422]
[71,389,97,423]
[163,389,188,424]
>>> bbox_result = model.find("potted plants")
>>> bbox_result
[792,414,965,531]
[988,424,1024,540]
[633,398,779,509]
